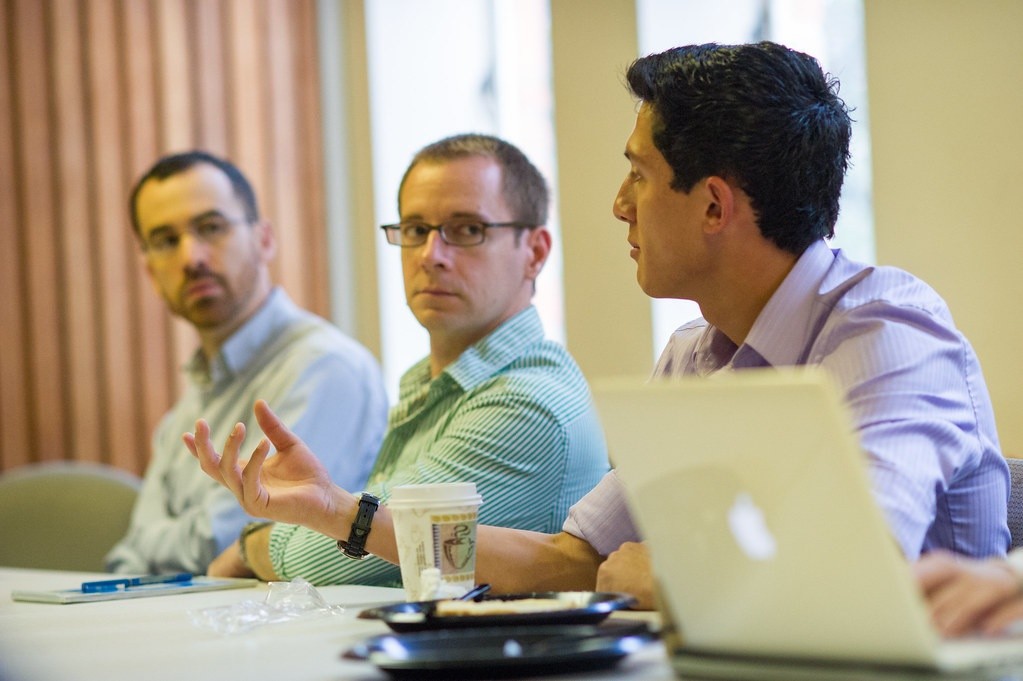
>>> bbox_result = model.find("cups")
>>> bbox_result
[386,483,484,601]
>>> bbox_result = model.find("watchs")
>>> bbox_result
[336,493,379,559]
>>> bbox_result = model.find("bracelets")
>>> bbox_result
[240,521,265,560]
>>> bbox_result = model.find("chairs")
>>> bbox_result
[0,461,143,573]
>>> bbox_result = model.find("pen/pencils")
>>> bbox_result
[81,572,195,594]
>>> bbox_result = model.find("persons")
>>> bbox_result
[184,40,1012,607]
[101,152,390,581]
[914,547,1023,636]
[207,133,611,598]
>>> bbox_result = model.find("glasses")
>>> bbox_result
[380,215,541,248]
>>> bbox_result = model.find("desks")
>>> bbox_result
[0,568,675,681]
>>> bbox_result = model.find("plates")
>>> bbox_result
[342,619,675,680]
[359,590,639,632]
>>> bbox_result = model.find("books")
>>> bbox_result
[12,577,258,605]
